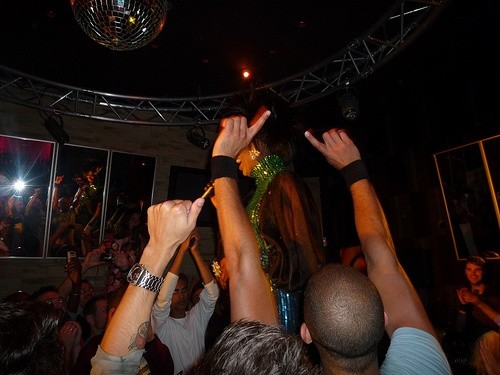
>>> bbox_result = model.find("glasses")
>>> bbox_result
[47,298,65,306]
[79,287,98,294]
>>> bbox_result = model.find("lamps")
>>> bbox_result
[339,92,358,122]
[43,115,68,145]
[187,127,210,151]
[71,0,167,51]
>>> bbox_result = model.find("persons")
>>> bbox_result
[90,197,322,375]
[216,119,327,336]
[0,176,150,257]
[0,245,230,375]
[77,171,102,217]
[210,110,456,375]
[442,256,500,375]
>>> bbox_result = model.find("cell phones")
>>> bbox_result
[67,251,76,264]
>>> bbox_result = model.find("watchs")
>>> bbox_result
[126,263,162,294]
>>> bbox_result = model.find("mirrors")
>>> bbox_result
[0,133,57,259]
[46,142,110,263]
[102,149,159,259]
[432,135,500,262]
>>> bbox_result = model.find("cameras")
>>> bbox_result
[101,252,115,262]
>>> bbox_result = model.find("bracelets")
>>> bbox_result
[340,160,369,186]
[210,155,238,178]
